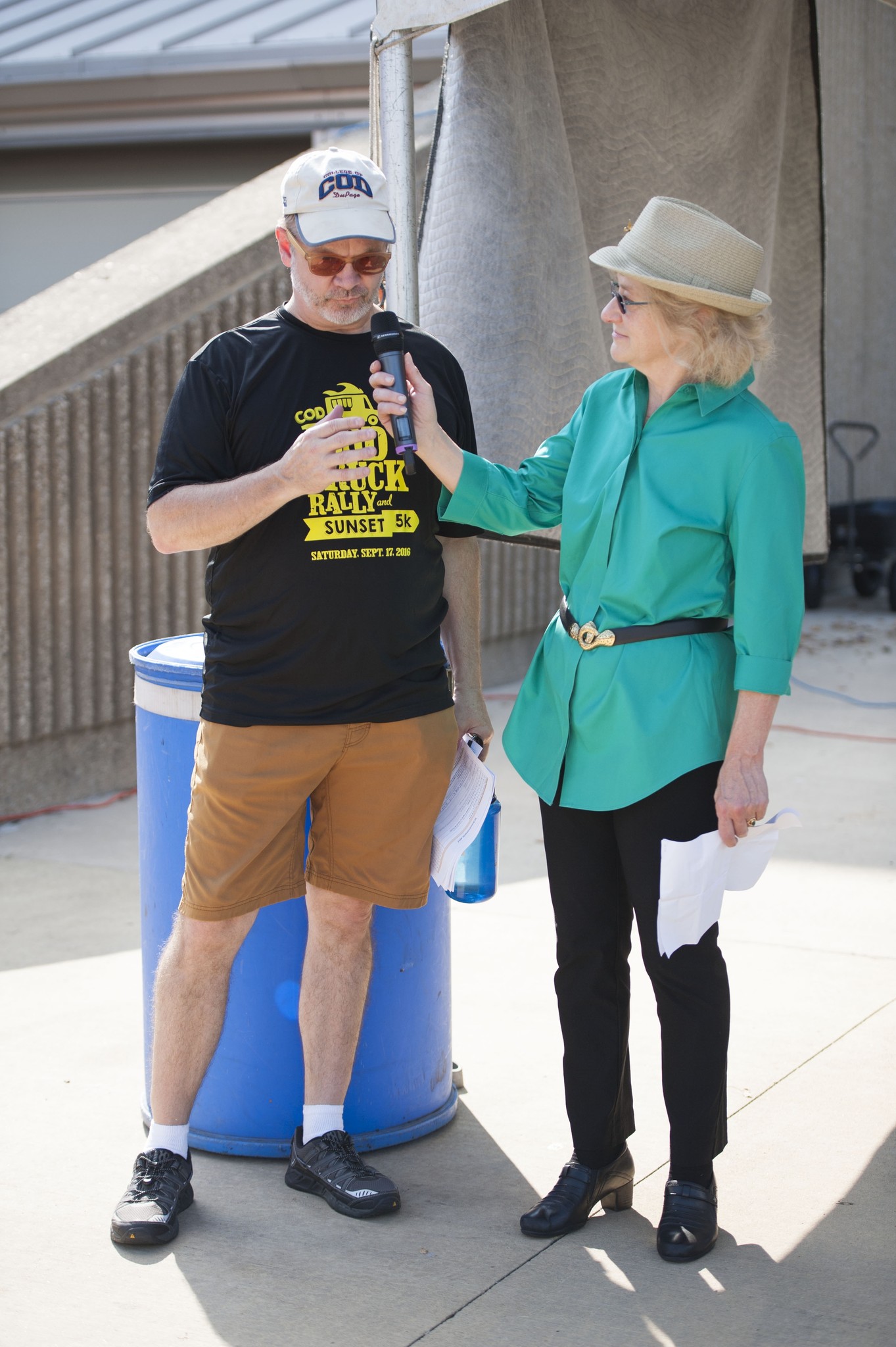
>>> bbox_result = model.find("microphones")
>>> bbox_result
[370,311,419,475]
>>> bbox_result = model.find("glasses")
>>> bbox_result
[283,227,391,276]
[610,281,661,314]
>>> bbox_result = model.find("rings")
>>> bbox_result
[747,818,755,827]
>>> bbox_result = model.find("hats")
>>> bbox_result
[281,146,396,244]
[588,196,772,316]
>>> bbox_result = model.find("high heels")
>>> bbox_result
[658,1173,718,1261]
[520,1145,634,1238]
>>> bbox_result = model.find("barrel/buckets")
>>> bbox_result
[128,631,461,1160]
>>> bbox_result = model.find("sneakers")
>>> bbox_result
[284,1126,402,1218]
[111,1147,194,1244]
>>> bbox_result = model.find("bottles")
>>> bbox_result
[445,788,501,904]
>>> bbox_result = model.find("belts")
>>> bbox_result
[559,594,734,650]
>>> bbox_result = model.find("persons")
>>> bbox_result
[106,147,478,1249]
[369,195,805,1263]
